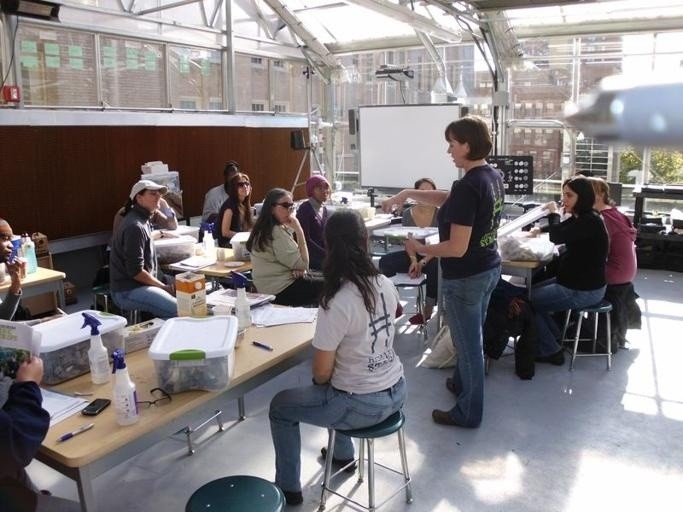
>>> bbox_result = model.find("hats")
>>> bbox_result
[306,176,327,197]
[129,179,168,201]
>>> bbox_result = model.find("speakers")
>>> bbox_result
[349,109,356,135]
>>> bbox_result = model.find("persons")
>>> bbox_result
[0,218,31,320]
[0,356,49,512]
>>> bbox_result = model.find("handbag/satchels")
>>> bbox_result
[568,315,618,355]
[420,325,457,368]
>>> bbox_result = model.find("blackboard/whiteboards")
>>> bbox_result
[358,103,462,191]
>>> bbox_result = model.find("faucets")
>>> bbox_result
[367,186,378,207]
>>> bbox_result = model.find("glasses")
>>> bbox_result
[276,202,294,208]
[137,388,171,410]
[238,181,250,187]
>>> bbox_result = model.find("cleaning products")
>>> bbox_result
[341,197,349,205]
[198,222,217,261]
[109,348,141,427]
[228,269,254,330]
[79,312,112,386]
[17,232,38,275]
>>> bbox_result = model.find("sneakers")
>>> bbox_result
[321,447,355,472]
[535,350,564,365]
[447,377,455,392]
[432,409,455,425]
[284,491,303,505]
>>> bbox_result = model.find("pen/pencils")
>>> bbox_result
[56,422,95,442]
[252,341,273,351]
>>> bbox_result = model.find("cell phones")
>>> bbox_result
[82,399,111,417]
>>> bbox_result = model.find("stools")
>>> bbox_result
[603,281,639,354]
[184,474,286,512]
[557,297,613,373]
[319,406,414,512]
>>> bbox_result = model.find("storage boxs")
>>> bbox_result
[140,171,181,191]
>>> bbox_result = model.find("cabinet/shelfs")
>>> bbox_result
[632,185,683,272]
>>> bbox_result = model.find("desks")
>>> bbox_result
[31,304,329,512]
[0,190,552,456]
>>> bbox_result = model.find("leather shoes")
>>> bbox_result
[409,312,431,324]
[396,308,402,318]
